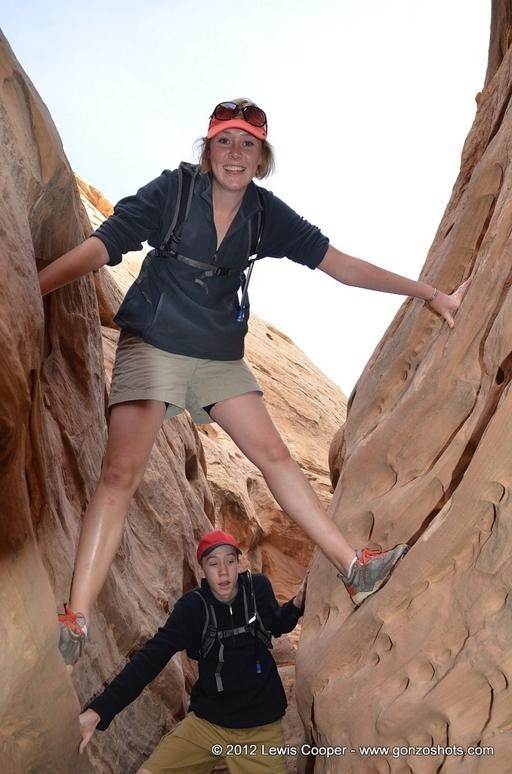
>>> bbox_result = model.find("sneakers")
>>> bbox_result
[340,543,413,607]
[58,600,88,666]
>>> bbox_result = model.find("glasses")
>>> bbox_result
[212,102,267,127]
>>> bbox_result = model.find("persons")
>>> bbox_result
[38,93,475,667]
[78,528,309,773]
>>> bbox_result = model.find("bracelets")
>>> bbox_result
[425,287,439,306]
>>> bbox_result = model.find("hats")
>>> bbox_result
[196,529,242,564]
[206,110,269,140]
